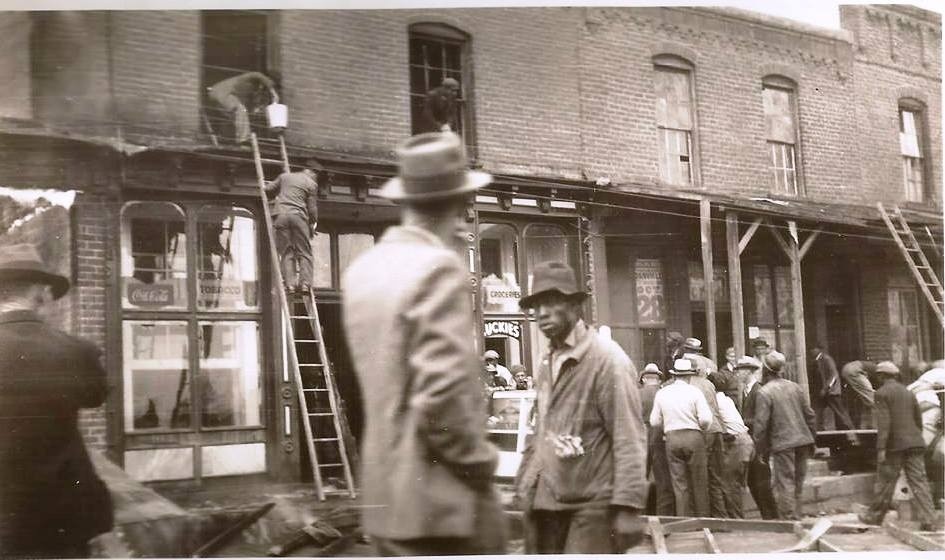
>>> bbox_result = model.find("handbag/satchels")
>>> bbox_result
[748,458,769,496]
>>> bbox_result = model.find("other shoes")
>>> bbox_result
[920,524,936,532]
[287,286,296,294]
[301,284,309,293]
[859,512,882,526]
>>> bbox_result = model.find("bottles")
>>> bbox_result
[527,376,532,389]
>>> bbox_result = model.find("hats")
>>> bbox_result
[0,243,69,299]
[484,366,498,374]
[806,341,824,348]
[876,361,899,375]
[266,70,281,89]
[518,262,591,308]
[753,337,770,347]
[639,363,662,384]
[738,356,760,369]
[484,350,499,361]
[442,78,460,90]
[685,338,703,350]
[668,359,696,375]
[305,159,324,175]
[761,351,786,374]
[668,332,684,343]
[374,131,493,200]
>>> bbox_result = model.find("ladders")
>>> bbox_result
[252,133,355,503]
[876,200,944,328]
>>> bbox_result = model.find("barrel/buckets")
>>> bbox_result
[264,99,289,131]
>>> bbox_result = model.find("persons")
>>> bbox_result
[263,160,319,294]
[207,64,282,143]
[423,78,460,132]
[513,260,650,553]
[480,350,531,391]
[639,329,945,530]
[338,131,511,555]
[0,242,115,559]
